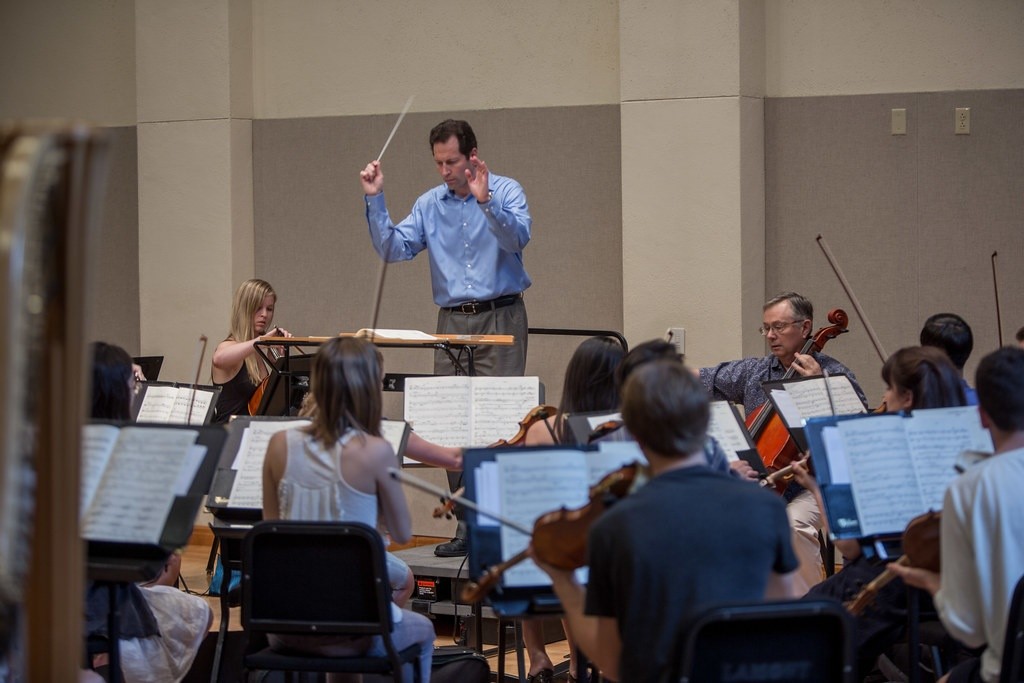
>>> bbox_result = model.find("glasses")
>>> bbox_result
[133,381,142,395]
[759,319,804,336]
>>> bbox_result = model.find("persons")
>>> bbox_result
[0,341,214,683]
[211,279,293,426]
[520,291,1024,683]
[262,335,463,683]
[359,119,532,556]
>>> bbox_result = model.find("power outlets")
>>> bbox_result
[956,108,971,135]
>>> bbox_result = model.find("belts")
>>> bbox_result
[441,293,521,315]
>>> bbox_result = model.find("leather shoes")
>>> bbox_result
[434,538,470,556]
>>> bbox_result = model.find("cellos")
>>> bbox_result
[245,327,290,416]
[743,306,853,489]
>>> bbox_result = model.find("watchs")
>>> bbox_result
[488,192,493,201]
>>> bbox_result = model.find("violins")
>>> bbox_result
[756,401,890,495]
[846,506,942,616]
[462,461,652,607]
[433,402,559,519]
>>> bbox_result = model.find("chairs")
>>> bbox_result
[204,502,1024,683]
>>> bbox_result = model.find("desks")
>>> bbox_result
[253,334,515,418]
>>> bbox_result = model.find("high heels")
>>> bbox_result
[567,672,589,683]
[527,669,554,683]
